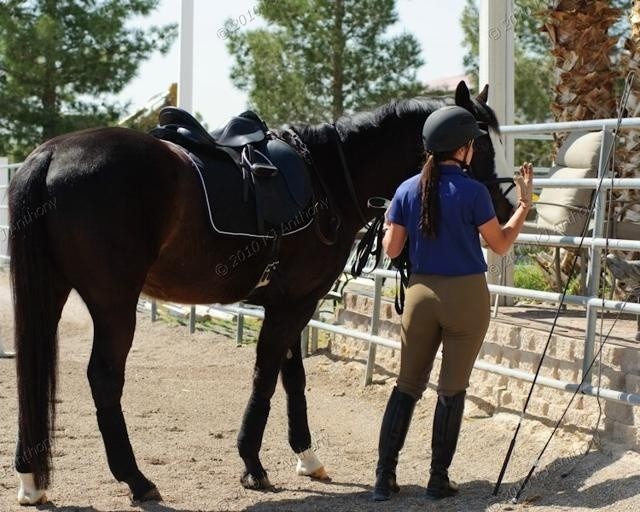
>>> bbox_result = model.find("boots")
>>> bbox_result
[374,385,415,501]
[426,390,466,499]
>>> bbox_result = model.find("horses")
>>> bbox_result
[6,75,514,508]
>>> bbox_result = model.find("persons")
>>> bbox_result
[368,104,536,502]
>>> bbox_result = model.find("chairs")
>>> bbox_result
[480,130,639,319]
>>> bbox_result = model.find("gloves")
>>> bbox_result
[513,162,533,207]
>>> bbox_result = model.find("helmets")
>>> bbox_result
[422,105,488,153]
